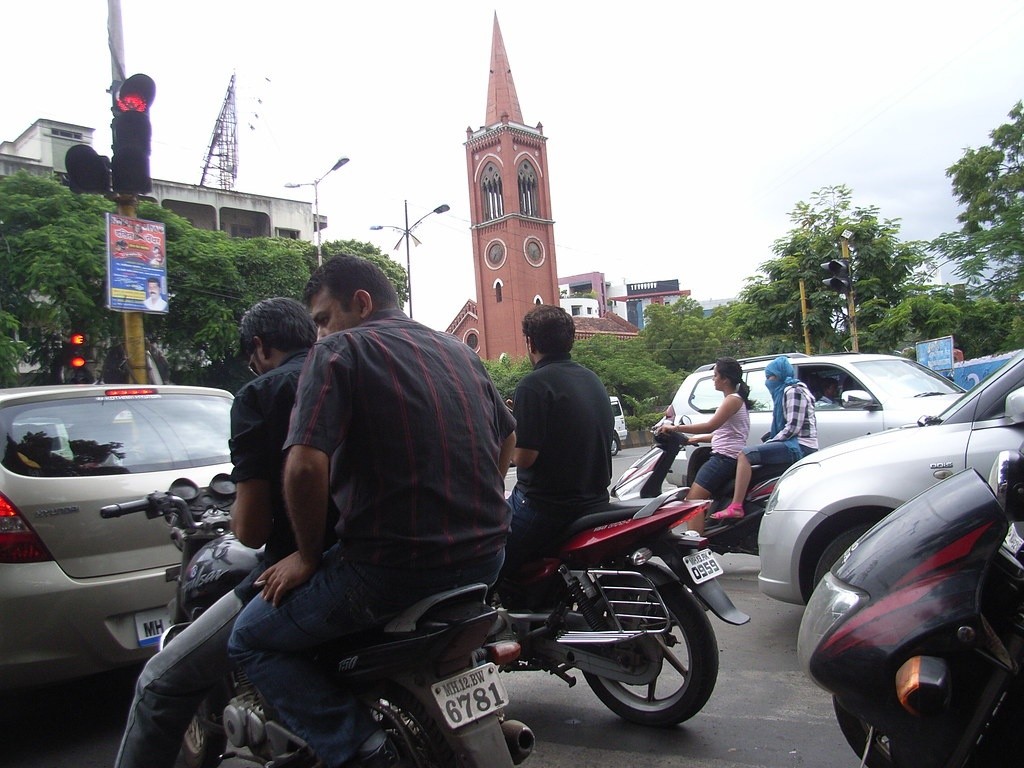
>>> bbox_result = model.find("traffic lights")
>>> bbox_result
[66,323,89,373]
[109,73,156,195]
[64,144,112,195]
[828,259,852,295]
[819,261,833,287]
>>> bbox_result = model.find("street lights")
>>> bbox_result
[284,157,350,267]
[370,198,451,319]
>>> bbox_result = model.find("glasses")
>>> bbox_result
[247,353,261,377]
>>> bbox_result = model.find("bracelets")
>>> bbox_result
[674,425,680,431]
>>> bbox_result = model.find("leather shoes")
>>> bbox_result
[263,734,401,768]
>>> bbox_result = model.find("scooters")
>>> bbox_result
[798,386,1024,768]
[607,411,795,556]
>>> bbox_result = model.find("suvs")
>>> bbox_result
[1,381,237,695]
[757,354,1024,606]
[665,351,970,487]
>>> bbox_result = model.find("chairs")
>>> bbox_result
[805,373,825,400]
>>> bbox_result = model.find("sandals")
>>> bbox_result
[710,503,744,520]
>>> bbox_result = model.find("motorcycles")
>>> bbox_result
[494,486,754,730]
[98,472,537,768]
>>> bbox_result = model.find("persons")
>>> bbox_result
[147,278,166,312]
[113,255,516,767]
[653,357,758,537]
[709,356,818,518]
[814,377,841,409]
[497,303,615,611]
